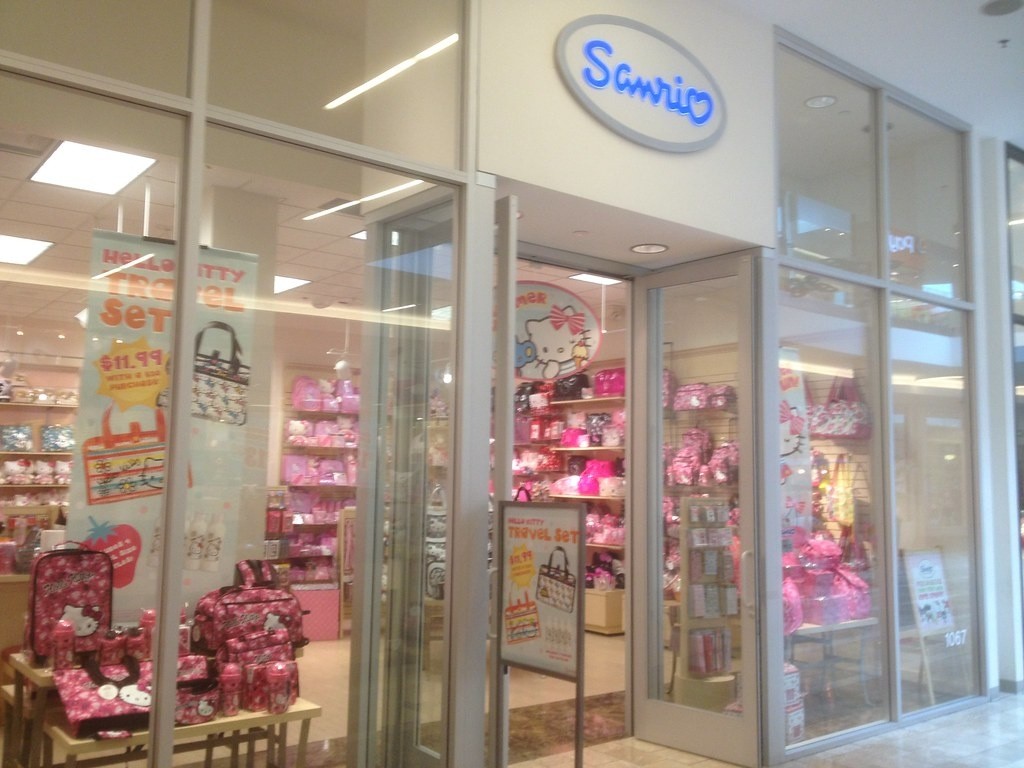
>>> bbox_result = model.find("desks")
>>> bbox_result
[782,616,876,713]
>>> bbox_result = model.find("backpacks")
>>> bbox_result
[782,527,872,635]
[292,375,360,414]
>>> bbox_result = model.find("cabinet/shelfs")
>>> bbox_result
[0,351,880,640]
[0,653,325,768]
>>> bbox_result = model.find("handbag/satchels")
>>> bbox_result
[425,485,447,600]
[662,368,740,598]
[805,377,871,527]
[0,528,43,574]
[52,650,221,740]
[193,560,304,703]
[512,368,626,589]
[23,541,113,660]
[282,454,356,485]
